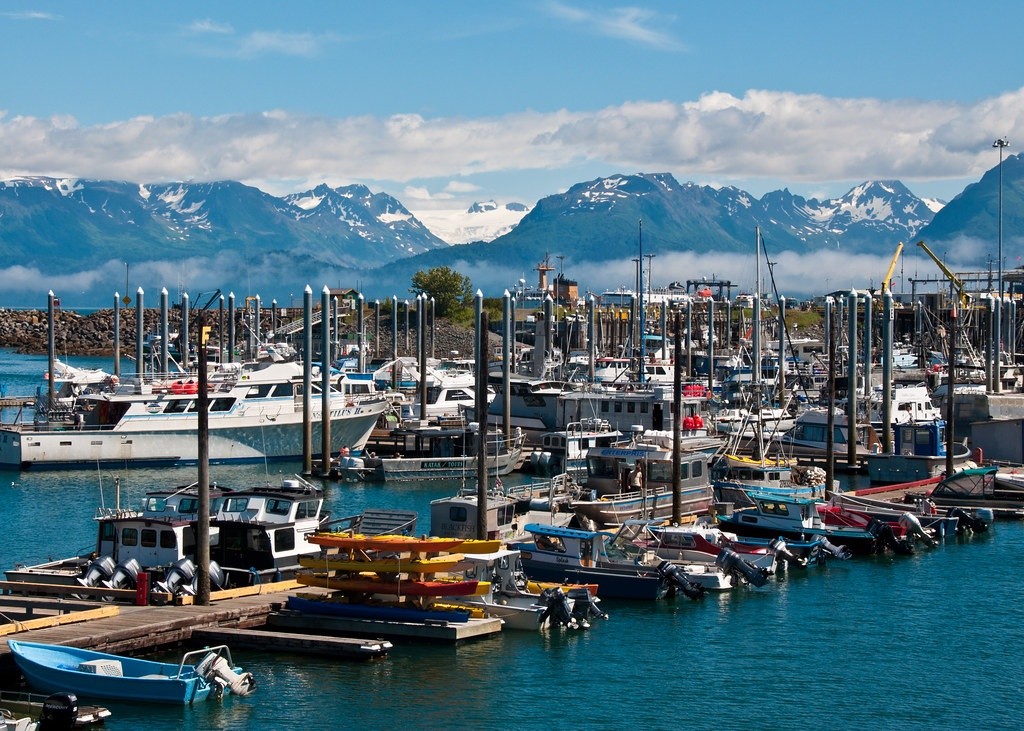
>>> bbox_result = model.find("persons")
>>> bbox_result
[339,446,401,459]
[627,466,642,495]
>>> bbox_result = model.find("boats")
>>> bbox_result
[7,638,257,707]
[1,217,1024,632]
